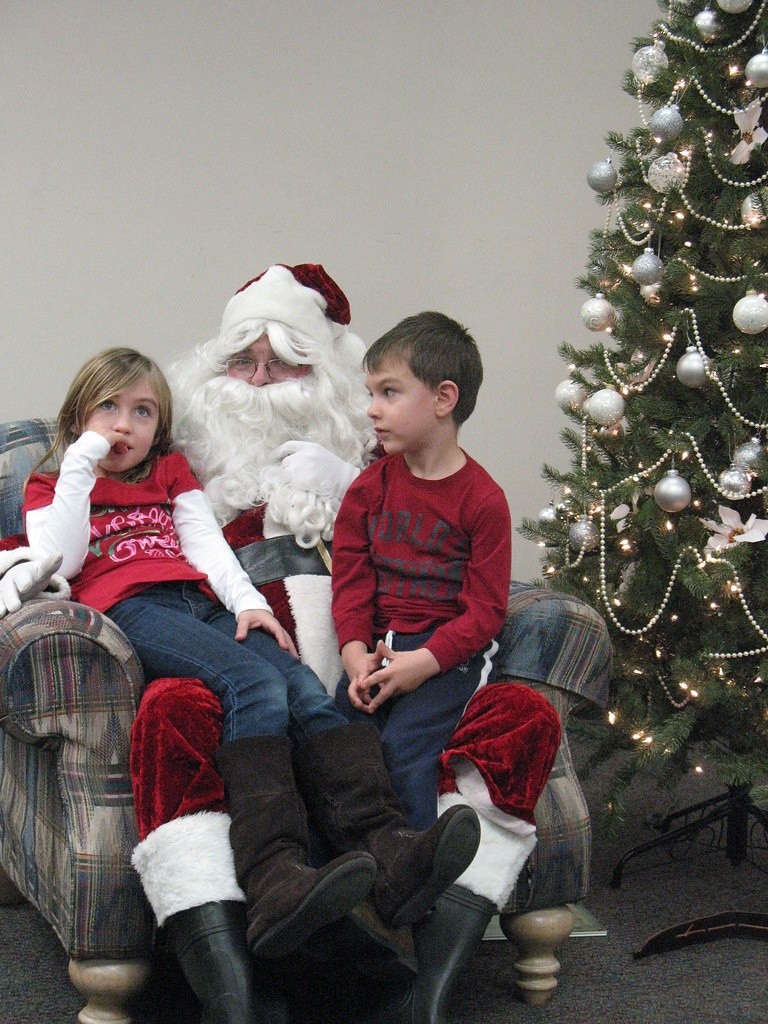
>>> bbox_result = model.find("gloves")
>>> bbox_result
[0,551,63,618]
[269,439,362,499]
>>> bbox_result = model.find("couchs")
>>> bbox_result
[0,412,621,1024]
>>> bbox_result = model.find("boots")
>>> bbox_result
[161,901,292,1024]
[291,724,483,927]
[355,884,498,1024]
[214,733,376,961]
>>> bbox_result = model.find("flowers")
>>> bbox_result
[723,95,768,166]
[698,503,768,555]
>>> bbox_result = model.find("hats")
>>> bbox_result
[220,263,368,366]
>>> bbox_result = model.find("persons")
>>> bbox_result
[25,347,481,957]
[333,312,511,978]
[0,265,564,1024]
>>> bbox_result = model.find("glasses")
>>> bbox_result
[225,353,299,382]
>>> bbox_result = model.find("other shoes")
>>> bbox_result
[338,902,420,982]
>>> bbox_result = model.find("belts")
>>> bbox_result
[235,525,338,587]
